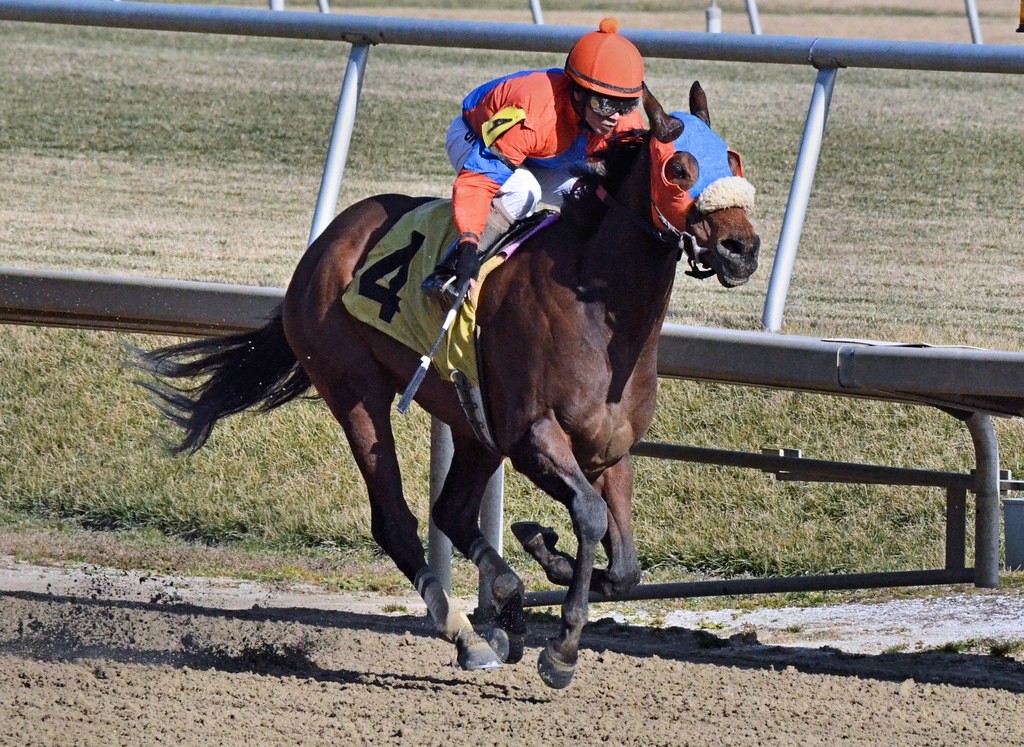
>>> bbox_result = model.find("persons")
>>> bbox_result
[420,19,647,306]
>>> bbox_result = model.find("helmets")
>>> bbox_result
[564,18,645,97]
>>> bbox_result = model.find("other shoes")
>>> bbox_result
[420,238,464,309]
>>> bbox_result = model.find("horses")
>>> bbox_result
[120,77,764,695]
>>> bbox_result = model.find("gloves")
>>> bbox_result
[454,240,480,293]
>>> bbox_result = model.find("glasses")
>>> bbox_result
[589,94,639,117]
